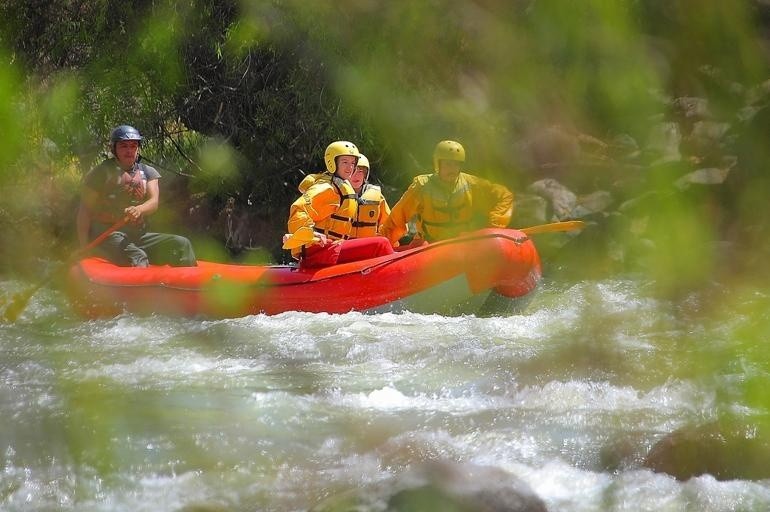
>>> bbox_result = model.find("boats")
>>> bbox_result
[63,222,543,320]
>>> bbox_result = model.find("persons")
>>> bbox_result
[342,154,409,252]
[375,138,517,252]
[67,123,200,271]
[280,138,397,272]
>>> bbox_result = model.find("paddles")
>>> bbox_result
[4,216,130,323]
[393,220,583,252]
[282,226,334,250]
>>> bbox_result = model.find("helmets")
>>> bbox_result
[325,141,370,181]
[432,140,466,174]
[110,125,143,154]
